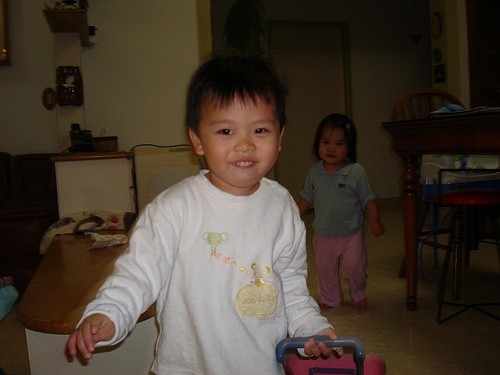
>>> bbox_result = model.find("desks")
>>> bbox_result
[14,228,159,375]
[381,113,500,312]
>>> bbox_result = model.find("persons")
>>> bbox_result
[293,113,384,311]
[68,55,342,375]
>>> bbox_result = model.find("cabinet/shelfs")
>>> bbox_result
[50,151,139,215]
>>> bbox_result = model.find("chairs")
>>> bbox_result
[391,87,464,278]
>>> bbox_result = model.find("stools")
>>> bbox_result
[435,188,500,324]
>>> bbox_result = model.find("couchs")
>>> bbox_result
[0,151,58,292]
